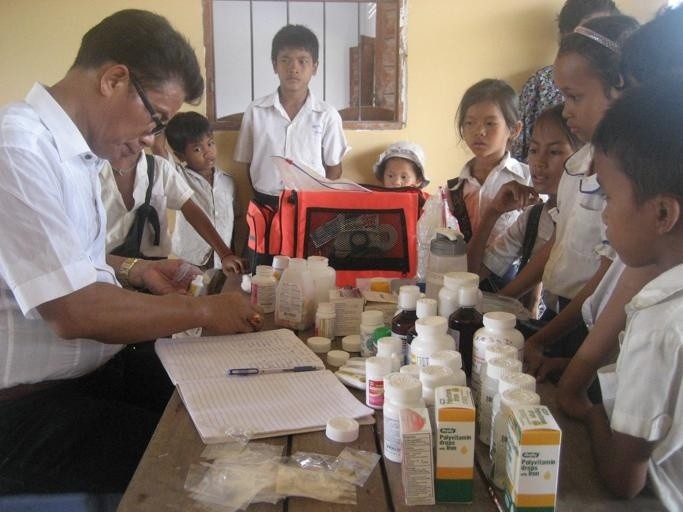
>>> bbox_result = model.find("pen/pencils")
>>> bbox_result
[228,365,325,376]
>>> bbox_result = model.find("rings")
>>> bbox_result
[248,313,263,327]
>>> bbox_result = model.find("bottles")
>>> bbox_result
[361,229,541,491]
[252,253,336,340]
[187,274,203,297]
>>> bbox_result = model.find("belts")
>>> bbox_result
[1,353,126,404]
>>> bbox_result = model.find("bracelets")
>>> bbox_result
[220,252,235,263]
[116,257,144,292]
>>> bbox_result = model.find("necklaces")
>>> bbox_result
[110,163,134,177]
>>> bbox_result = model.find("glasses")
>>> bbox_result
[112,52,171,137]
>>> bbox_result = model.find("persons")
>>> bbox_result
[584,64,682,511]
[95,147,253,295]
[146,110,245,276]
[1,8,267,501]
[232,23,352,270]
[440,75,544,320]
[524,6,682,422]
[465,102,590,322]
[506,0,624,165]
[370,140,434,195]
[483,14,644,409]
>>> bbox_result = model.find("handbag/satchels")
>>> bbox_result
[244,156,429,290]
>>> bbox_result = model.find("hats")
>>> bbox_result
[373,139,430,190]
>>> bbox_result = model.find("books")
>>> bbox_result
[148,327,377,444]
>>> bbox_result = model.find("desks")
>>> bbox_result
[114,271,667,512]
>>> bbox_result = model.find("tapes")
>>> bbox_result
[325,416,360,444]
